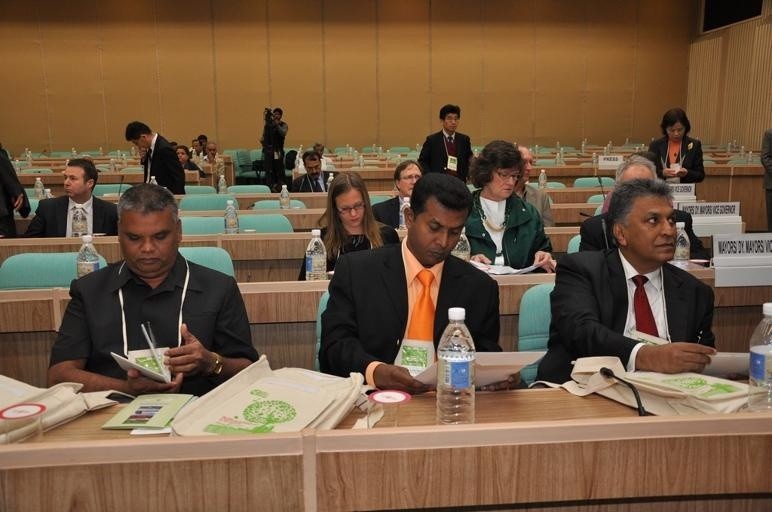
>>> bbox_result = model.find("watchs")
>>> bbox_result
[207,353,224,377]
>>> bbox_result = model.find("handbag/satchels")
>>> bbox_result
[570,355,769,416]
[170,354,384,451]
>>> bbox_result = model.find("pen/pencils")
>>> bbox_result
[694,330,703,344]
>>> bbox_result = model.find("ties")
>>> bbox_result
[631,275,659,344]
[73,205,87,237]
[408,269,435,340]
[448,135,453,142]
[314,178,322,192]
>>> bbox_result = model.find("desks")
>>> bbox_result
[0,268,772,388]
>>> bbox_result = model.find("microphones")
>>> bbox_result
[579,212,594,218]
[598,365,648,417]
[598,174,606,204]
[600,219,610,251]
[117,174,125,196]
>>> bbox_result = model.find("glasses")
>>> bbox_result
[495,170,521,181]
[335,201,365,214]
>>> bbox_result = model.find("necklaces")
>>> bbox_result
[478,194,509,231]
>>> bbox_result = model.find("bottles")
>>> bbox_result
[295,143,425,172]
[34,177,45,199]
[218,175,228,194]
[672,222,691,270]
[436,307,475,425]
[726,139,754,165]
[224,200,240,234]
[748,302,772,414]
[76,235,100,280]
[150,176,157,185]
[15,147,140,175]
[451,226,471,263]
[279,185,290,209]
[514,138,655,176]
[538,170,547,189]
[306,230,327,281]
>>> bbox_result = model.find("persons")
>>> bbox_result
[578,155,711,267]
[20,158,118,236]
[48,183,259,395]
[372,160,424,229]
[647,108,705,183]
[419,104,473,185]
[258,106,339,192]
[463,140,557,273]
[0,142,31,238]
[127,121,224,193]
[298,172,400,280]
[761,128,772,232]
[319,173,521,394]
[535,178,719,382]
[514,144,554,226]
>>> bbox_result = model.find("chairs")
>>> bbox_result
[518,284,556,385]
[314,289,330,371]
